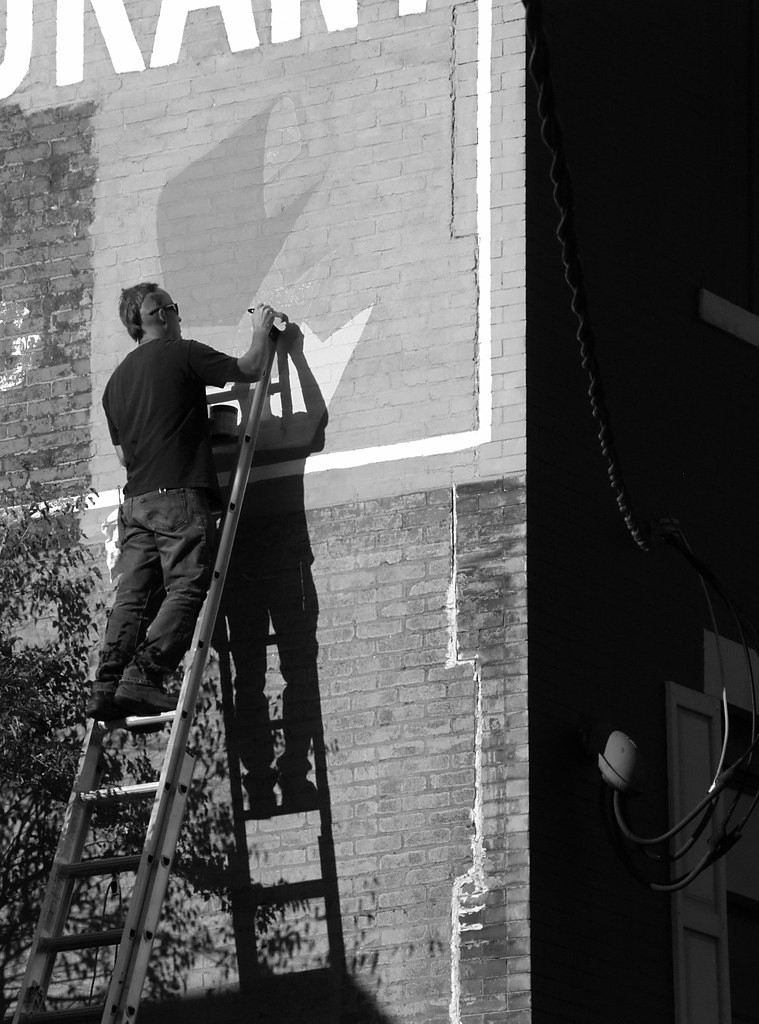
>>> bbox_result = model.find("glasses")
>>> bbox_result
[148,303,179,316]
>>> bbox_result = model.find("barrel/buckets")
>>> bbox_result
[210,405,238,436]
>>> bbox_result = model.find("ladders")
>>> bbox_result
[11,322,282,1024]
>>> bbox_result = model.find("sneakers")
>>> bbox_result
[86,691,164,734]
[113,681,179,711]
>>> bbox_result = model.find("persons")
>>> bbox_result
[87,282,281,731]
